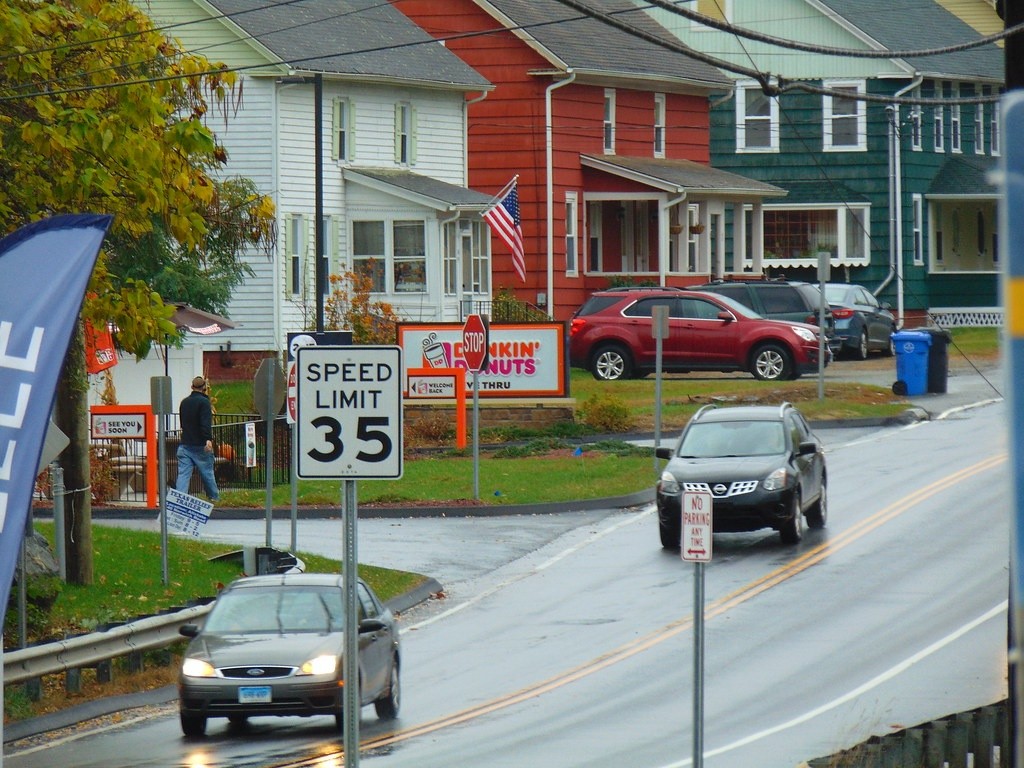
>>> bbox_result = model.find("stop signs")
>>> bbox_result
[286,361,295,425]
[462,314,486,371]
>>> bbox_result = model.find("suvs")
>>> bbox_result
[570,285,833,381]
[706,279,842,355]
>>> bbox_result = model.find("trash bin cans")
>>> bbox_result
[913,326,952,395]
[888,330,933,395]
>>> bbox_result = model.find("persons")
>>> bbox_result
[175,375,222,503]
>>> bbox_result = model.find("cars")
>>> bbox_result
[178,572,400,735]
[814,283,896,360]
[655,402,828,548]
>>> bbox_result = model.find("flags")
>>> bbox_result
[480,182,528,284]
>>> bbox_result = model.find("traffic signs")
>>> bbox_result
[681,491,713,563]
